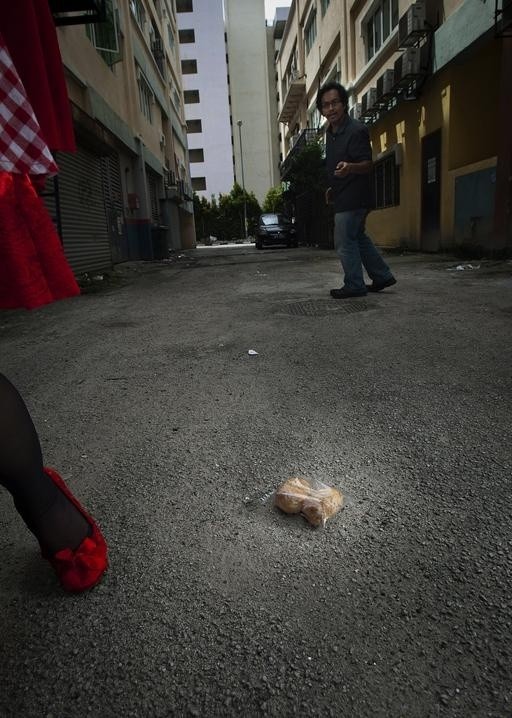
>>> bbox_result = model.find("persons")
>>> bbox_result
[314,80,397,298]
[2,1,108,596]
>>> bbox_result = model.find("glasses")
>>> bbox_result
[321,100,339,109]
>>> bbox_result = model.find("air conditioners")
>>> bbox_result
[347,2,427,122]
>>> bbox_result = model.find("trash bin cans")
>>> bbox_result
[152,225,169,260]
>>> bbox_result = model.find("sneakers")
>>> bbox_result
[331,286,366,299]
[32,468,108,593]
[366,275,397,291]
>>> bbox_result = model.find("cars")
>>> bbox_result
[256,211,298,248]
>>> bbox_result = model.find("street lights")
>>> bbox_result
[237,119,249,238]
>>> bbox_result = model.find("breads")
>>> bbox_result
[275,478,344,526]
